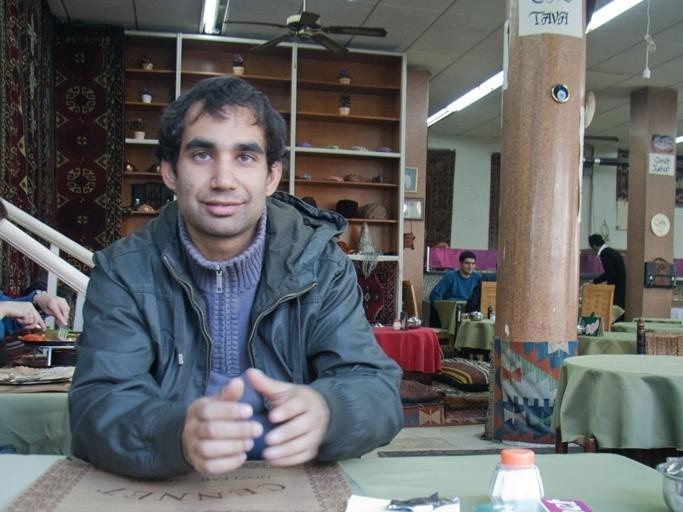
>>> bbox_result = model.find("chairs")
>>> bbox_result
[480,282,496,317]
[581,283,615,333]
[635,319,682,356]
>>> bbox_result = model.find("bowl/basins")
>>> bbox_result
[658,461,683,509]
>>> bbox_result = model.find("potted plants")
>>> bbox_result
[130,51,353,141]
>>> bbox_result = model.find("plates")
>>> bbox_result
[651,213,670,237]
[12,329,79,346]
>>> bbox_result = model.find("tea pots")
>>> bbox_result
[469,310,483,321]
[407,316,423,327]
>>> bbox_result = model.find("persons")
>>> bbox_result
[0,199,72,340]
[578,233,625,325]
[65,74,407,481]
[428,249,485,306]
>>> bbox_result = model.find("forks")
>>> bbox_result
[58,321,69,341]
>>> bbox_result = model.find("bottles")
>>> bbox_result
[489,448,542,500]
[125,158,133,171]
[487,304,493,318]
[392,320,402,330]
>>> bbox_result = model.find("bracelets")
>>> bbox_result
[33,290,48,306]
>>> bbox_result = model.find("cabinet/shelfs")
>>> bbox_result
[117,26,408,324]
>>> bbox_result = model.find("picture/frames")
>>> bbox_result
[402,196,424,222]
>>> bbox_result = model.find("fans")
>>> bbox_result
[218,0,388,59]
